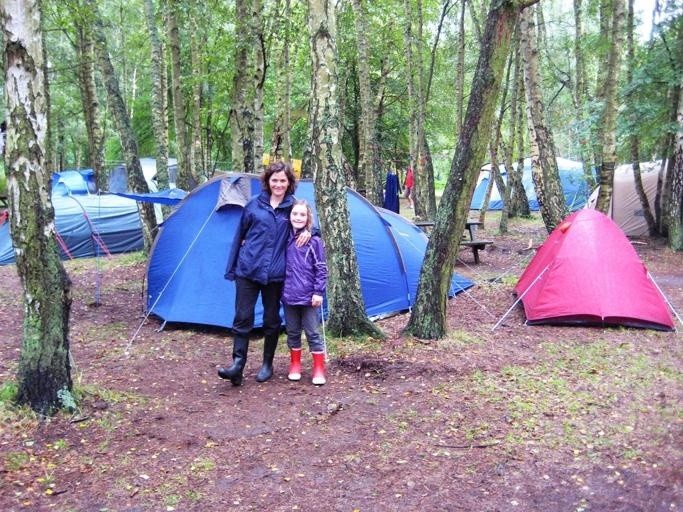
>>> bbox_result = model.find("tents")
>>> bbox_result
[510,209,675,334]
[469,155,682,246]
[0,158,203,265]
[142,172,478,335]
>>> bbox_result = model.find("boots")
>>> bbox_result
[257,335,278,382]
[288,349,302,381]
[311,351,326,385]
[217,336,248,384]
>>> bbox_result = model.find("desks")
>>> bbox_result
[415,220,484,264]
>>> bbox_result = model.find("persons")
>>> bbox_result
[216,158,321,389]
[402,166,415,210]
[241,198,327,386]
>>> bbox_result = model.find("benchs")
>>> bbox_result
[460,240,493,264]
